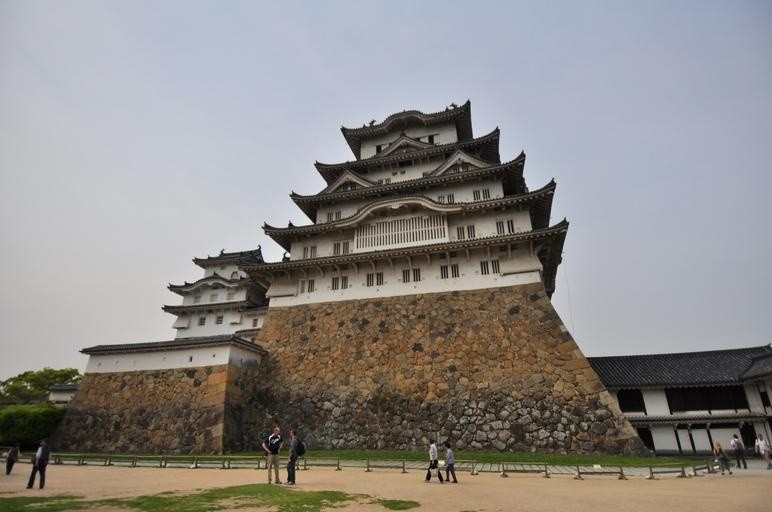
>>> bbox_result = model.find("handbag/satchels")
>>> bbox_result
[429,467,440,478]
[32,454,36,464]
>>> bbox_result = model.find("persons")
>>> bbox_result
[443,442,458,484]
[730,433,748,469]
[713,441,732,475]
[261,426,284,486]
[286,430,300,486]
[5,442,22,475]
[26,440,50,489]
[425,438,446,485]
[753,432,771,470]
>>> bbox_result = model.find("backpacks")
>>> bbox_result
[295,437,306,457]
